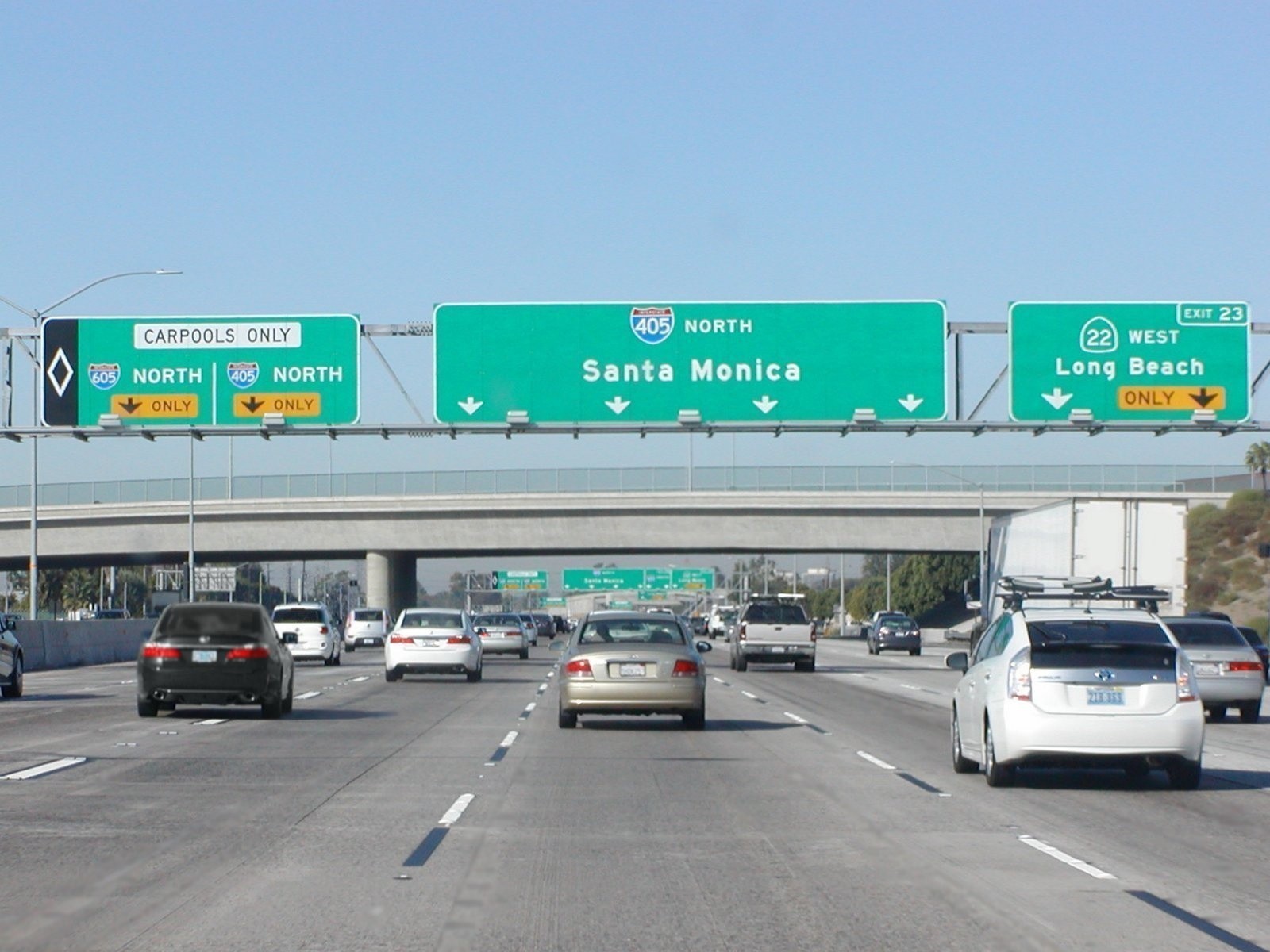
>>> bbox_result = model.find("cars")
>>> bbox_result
[342,608,397,653]
[1157,609,1270,724]
[94,608,131,620]
[133,599,299,720]
[0,611,26,699]
[866,610,921,656]
[546,609,713,732]
[382,606,488,683]
[517,606,741,648]
[472,612,529,659]
[270,600,343,667]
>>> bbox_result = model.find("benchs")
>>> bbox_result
[411,620,457,628]
[480,620,515,627]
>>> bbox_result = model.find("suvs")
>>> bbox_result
[943,572,1207,791]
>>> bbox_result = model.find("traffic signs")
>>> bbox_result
[39,312,361,429]
[1009,300,1253,429]
[433,298,948,429]
[644,567,716,591]
[490,570,549,591]
[561,567,646,592]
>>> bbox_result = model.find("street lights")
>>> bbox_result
[0,269,184,620]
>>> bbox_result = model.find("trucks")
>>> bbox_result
[963,494,1189,644]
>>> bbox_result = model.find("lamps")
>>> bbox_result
[98,413,123,430]
[678,409,702,424]
[505,410,530,425]
[1191,410,1215,422]
[263,413,288,428]
[1070,408,1093,423]
[853,408,878,424]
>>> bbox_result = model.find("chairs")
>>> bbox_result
[178,620,201,633]
[649,632,671,642]
[240,620,253,635]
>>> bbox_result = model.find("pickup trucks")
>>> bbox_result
[727,592,817,672]
